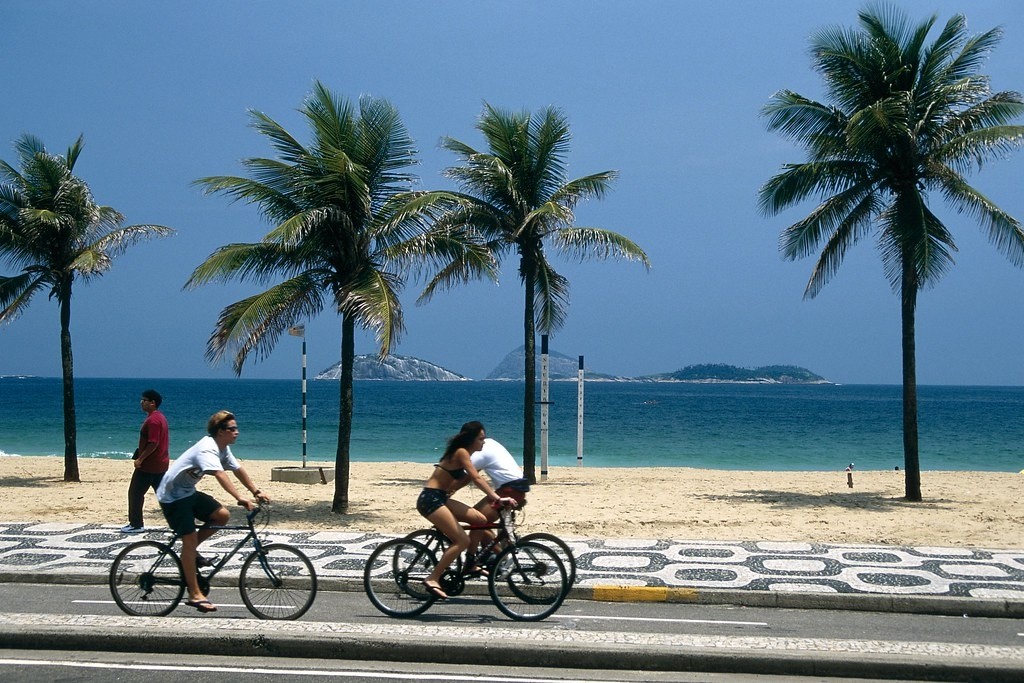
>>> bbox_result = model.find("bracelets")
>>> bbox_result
[253,489,261,497]
[496,497,500,503]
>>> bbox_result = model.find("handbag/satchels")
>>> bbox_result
[501,478,529,492]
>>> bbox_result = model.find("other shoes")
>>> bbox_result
[497,555,518,580]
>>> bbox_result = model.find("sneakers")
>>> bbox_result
[121,523,145,532]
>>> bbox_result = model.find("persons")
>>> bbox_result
[845,463,854,471]
[446,438,530,579]
[121,390,170,532]
[156,409,271,612]
[417,421,519,600]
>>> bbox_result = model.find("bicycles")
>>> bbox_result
[363,497,568,621]
[109,497,318,621]
[394,497,576,604]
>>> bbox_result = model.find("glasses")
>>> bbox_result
[223,427,237,432]
[140,399,149,403]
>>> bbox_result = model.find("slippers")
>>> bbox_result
[464,571,489,578]
[180,555,211,566]
[185,601,216,611]
[422,581,446,600]
